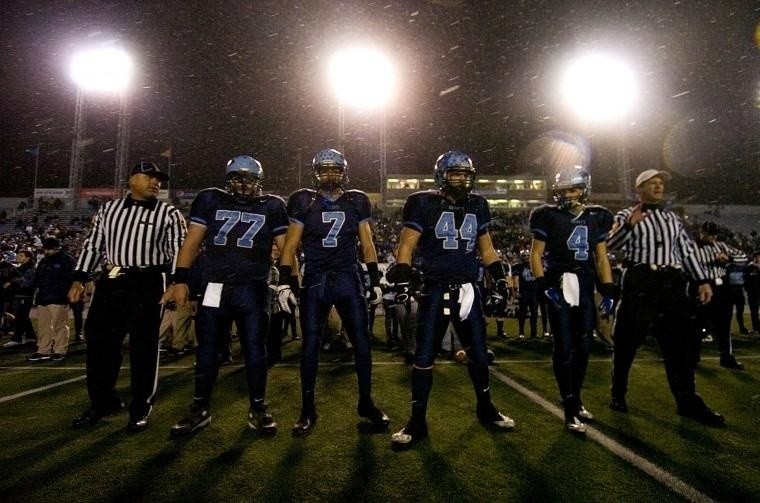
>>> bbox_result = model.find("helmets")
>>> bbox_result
[433,151,478,201]
[552,166,591,210]
[224,155,264,199]
[312,149,350,192]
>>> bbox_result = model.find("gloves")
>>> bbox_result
[268,284,281,314]
[394,282,411,314]
[278,284,297,314]
[538,287,562,310]
[598,295,616,320]
[365,286,383,304]
[483,279,508,318]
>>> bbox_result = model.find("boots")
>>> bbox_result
[720,355,744,369]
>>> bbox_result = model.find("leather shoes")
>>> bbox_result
[73,402,125,429]
[126,419,149,436]
[676,398,724,424]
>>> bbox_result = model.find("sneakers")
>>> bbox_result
[476,403,516,429]
[247,403,277,433]
[25,353,51,361]
[3,341,21,347]
[170,403,211,437]
[609,395,628,412]
[559,396,594,432]
[391,418,428,448]
[293,406,316,433]
[51,353,65,361]
[159,344,190,357]
[357,402,391,426]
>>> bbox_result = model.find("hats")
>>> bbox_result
[702,221,720,235]
[129,161,169,182]
[635,168,672,188]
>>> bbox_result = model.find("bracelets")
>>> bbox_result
[173,265,189,285]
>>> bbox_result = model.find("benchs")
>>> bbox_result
[1,206,98,237]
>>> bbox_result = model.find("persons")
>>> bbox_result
[390,150,517,443]
[1,197,106,362]
[158,199,205,362]
[320,210,423,355]
[269,243,300,340]
[589,254,625,352]
[529,162,619,435]
[678,206,760,369]
[68,159,187,431]
[604,167,725,425]
[439,206,552,362]
[171,155,300,438]
[277,148,390,432]
